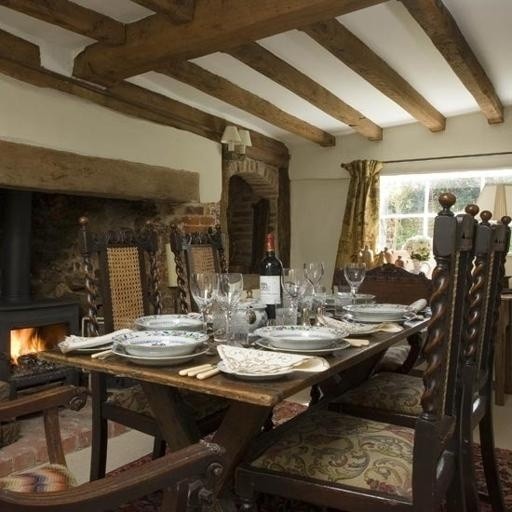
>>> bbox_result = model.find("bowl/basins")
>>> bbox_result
[111,314,210,367]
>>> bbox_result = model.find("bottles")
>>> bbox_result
[260,234,283,318]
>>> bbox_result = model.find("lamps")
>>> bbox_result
[221,125,252,155]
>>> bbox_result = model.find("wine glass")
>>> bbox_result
[282,262,368,326]
[190,271,244,354]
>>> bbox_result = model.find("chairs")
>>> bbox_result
[0,193,511,511]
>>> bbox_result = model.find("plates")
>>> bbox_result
[72,347,112,355]
[217,304,417,378]
